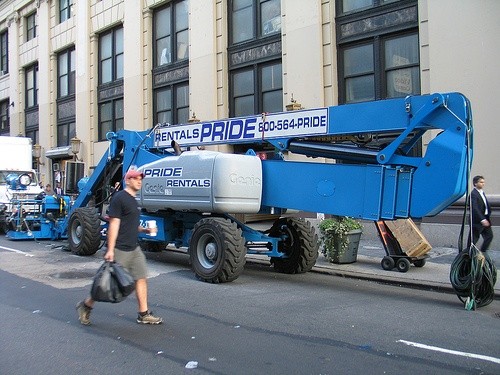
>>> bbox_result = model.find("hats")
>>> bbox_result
[125,170,144,180]
[46,184,51,187]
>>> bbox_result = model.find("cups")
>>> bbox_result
[147,220,158,237]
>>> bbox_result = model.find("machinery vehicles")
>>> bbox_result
[7,91,474,284]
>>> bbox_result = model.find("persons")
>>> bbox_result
[75,170,165,326]
[53,183,75,197]
[108,182,120,203]
[35,184,58,205]
[467,176,494,253]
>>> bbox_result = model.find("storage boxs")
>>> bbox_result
[384,217,432,259]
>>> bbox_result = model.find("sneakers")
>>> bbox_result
[76,300,93,326]
[136,310,162,324]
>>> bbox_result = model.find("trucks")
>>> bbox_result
[0,136,46,214]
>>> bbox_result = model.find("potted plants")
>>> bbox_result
[316,215,363,264]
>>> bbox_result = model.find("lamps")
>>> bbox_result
[70,136,83,162]
[188,112,205,150]
[32,144,45,166]
[286,93,304,111]
